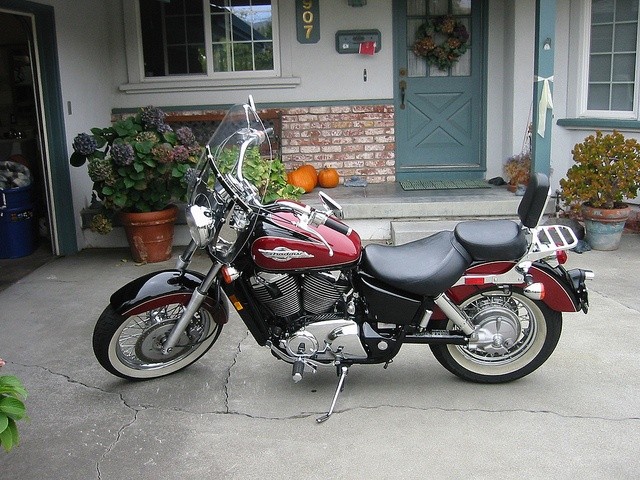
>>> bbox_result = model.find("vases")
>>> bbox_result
[118,206,176,263]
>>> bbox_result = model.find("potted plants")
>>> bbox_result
[559,129,639,250]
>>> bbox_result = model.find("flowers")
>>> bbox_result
[412,15,472,72]
[70,106,201,234]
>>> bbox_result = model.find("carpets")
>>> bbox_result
[399,178,492,190]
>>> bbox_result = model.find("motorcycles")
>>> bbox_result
[93,95,594,424]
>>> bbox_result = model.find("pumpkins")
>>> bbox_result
[297,165,318,187]
[319,167,339,188]
[286,169,315,193]
